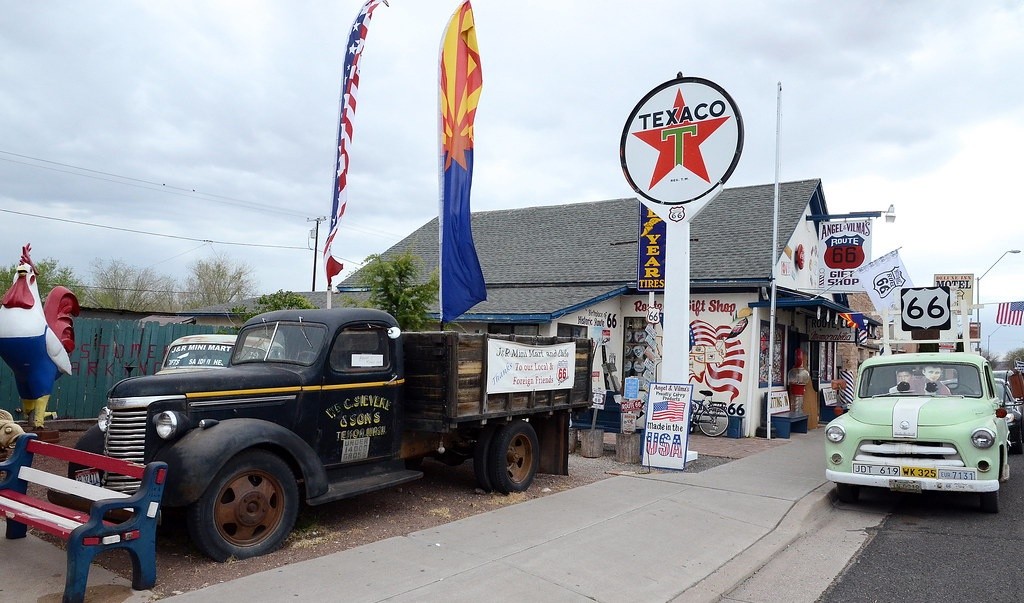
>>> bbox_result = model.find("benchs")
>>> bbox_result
[771,411,809,439]
[820,387,850,423]
[0,432,168,602]
[570,390,645,455]
[691,412,746,439]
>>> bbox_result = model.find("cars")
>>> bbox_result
[822,350,1012,514]
[989,378,1023,457]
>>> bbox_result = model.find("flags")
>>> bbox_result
[856,250,914,321]
[437,0,487,323]
[321,0,391,284]
[996,301,1024,326]
[840,312,869,332]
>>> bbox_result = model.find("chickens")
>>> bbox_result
[0,243,81,430]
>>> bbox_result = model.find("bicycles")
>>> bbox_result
[692,389,729,437]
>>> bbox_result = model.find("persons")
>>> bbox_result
[907,365,951,395]
[890,367,915,393]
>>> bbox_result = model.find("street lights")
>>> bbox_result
[975,250,1019,353]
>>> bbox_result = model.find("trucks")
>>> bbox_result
[69,306,593,571]
